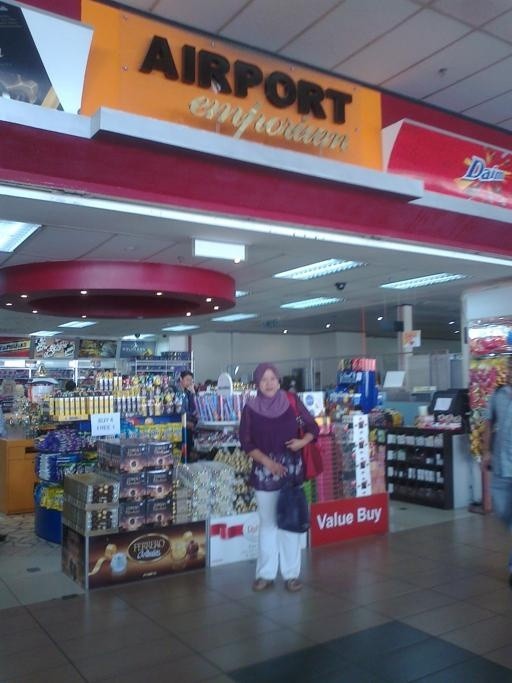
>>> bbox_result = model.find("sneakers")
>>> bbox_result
[286,579,302,592]
[253,578,273,592]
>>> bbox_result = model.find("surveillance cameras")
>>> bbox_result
[335,282,345,290]
[163,335,167,337]
[135,333,141,338]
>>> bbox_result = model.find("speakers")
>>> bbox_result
[393,321,404,333]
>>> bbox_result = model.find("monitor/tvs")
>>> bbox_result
[429,389,465,416]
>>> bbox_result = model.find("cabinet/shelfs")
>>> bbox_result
[0,332,475,593]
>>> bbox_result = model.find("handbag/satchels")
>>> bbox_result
[300,433,321,480]
[486,433,497,471]
[277,476,310,532]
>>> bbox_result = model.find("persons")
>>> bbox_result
[481,357,512,592]
[177,370,198,454]
[239,362,319,593]
[65,381,77,392]
